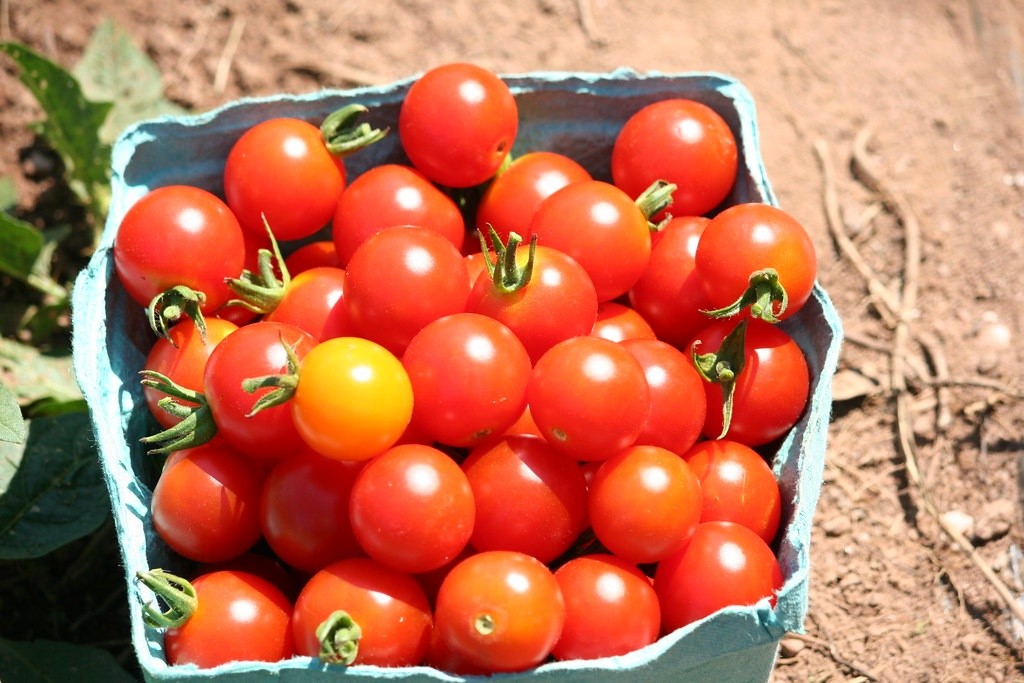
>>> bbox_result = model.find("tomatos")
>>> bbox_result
[113,64,816,671]
